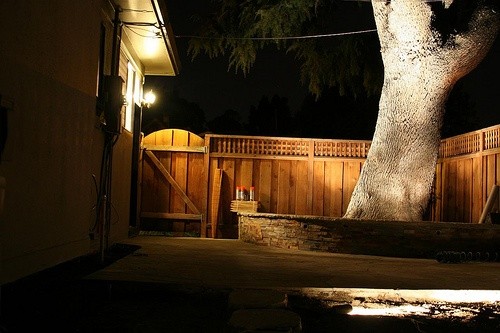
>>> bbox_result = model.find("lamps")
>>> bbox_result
[141,90,157,108]
